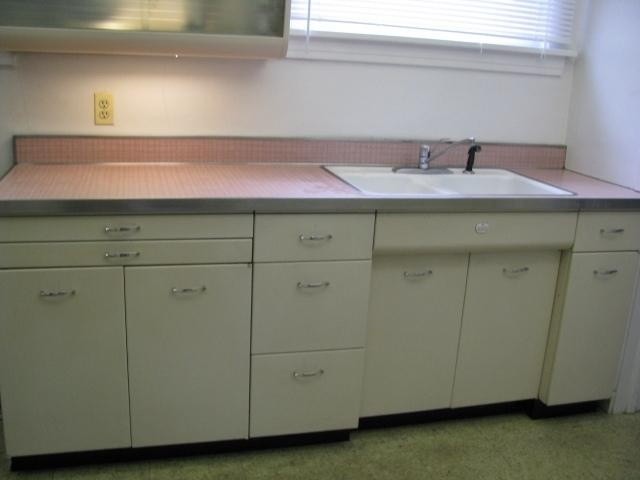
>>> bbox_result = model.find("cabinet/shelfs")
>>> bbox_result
[0,211,640,474]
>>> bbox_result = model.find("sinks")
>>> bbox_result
[320,164,447,198]
[414,167,577,196]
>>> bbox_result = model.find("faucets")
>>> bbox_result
[418,137,475,170]
